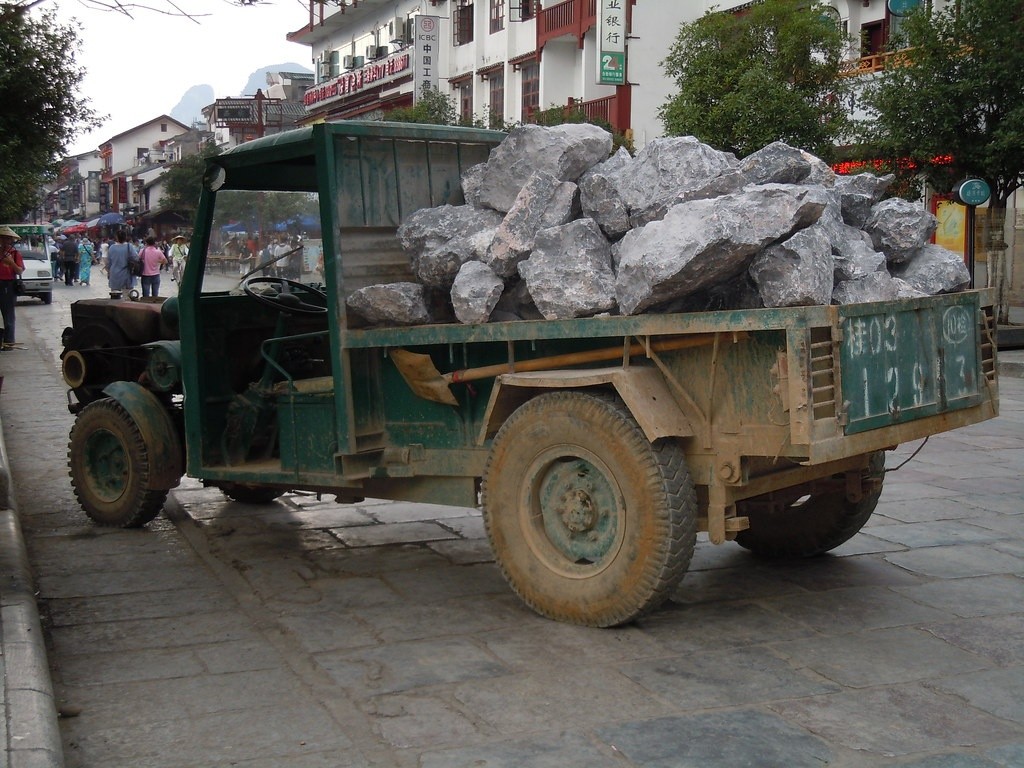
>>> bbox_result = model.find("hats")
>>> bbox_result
[171,234,187,242]
[0,226,21,239]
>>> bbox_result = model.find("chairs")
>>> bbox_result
[259,331,342,474]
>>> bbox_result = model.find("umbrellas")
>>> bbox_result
[35,215,131,236]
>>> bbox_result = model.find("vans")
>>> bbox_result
[1,224,54,304]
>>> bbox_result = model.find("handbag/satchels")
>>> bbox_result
[16,279,26,296]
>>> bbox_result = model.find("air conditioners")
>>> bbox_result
[389,16,402,44]
[365,44,377,58]
[319,48,330,64]
[343,54,354,69]
[319,63,329,78]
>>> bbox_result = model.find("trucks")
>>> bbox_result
[53,117,1002,630]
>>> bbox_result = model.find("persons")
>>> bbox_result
[134,238,167,299]
[47,239,58,277]
[55,239,64,281]
[159,241,170,273]
[223,225,308,281]
[63,234,77,285]
[167,234,191,290]
[105,230,142,299]
[99,239,109,278]
[35,230,197,254]
[1,225,26,351]
[78,237,98,286]
